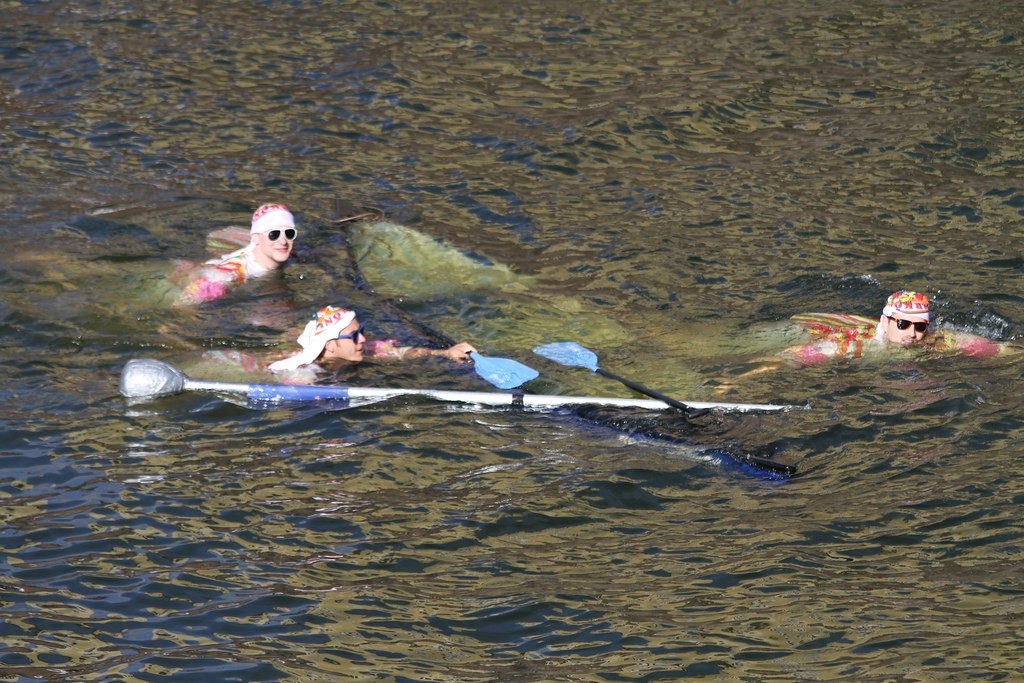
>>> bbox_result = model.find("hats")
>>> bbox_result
[886,289,930,314]
[250,203,295,234]
[315,306,356,335]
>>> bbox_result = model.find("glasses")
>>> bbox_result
[263,228,298,242]
[339,323,366,345]
[887,316,930,332]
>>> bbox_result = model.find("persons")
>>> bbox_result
[249,204,297,270]
[878,290,930,346]
[299,305,477,385]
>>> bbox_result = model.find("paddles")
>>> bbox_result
[533,337,798,476]
[370,295,541,390]
